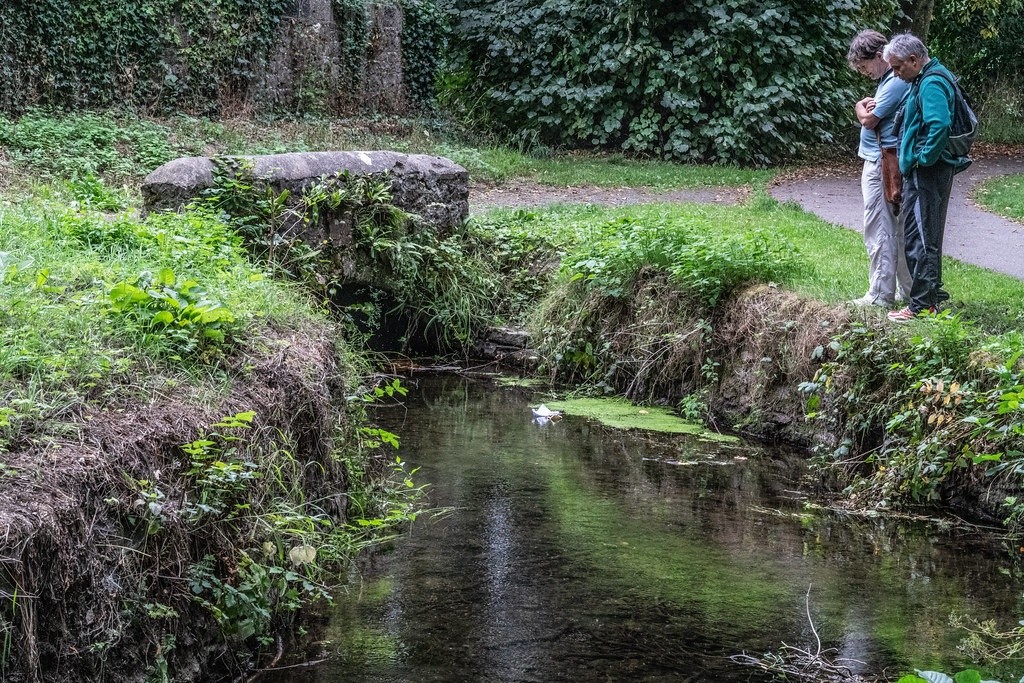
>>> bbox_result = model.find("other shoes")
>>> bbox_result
[848,298,878,310]
[887,305,937,323]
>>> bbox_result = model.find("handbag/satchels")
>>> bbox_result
[881,146,903,204]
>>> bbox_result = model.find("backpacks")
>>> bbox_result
[917,70,978,156]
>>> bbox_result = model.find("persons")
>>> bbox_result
[883,34,960,326]
[848,30,914,307]
